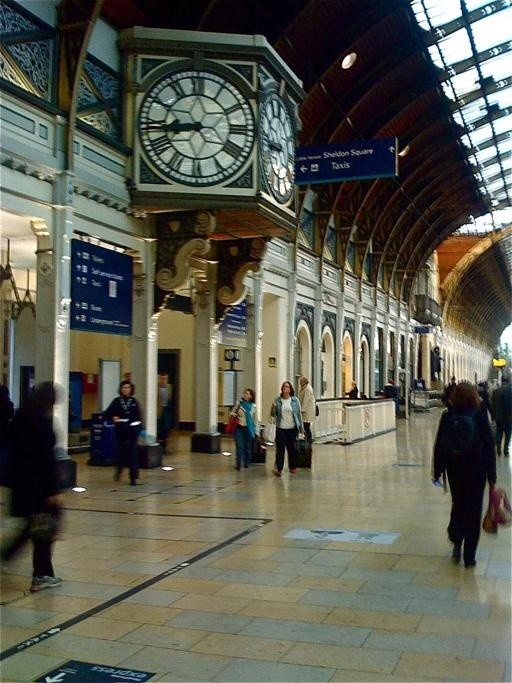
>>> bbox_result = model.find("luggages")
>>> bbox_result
[295,430,311,468]
[251,435,266,463]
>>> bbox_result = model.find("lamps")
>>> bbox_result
[327,43,359,72]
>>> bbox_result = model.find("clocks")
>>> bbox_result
[258,63,297,218]
[134,50,258,196]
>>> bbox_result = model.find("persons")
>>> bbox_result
[298,376,317,467]
[106,382,144,485]
[270,381,305,475]
[342,375,510,457]
[2,380,65,592]
[124,370,133,381]
[159,373,174,452]
[230,387,260,470]
[431,380,496,566]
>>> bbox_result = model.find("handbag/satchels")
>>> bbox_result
[483,488,511,534]
[227,416,239,433]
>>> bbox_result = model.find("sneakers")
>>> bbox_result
[30,575,63,591]
